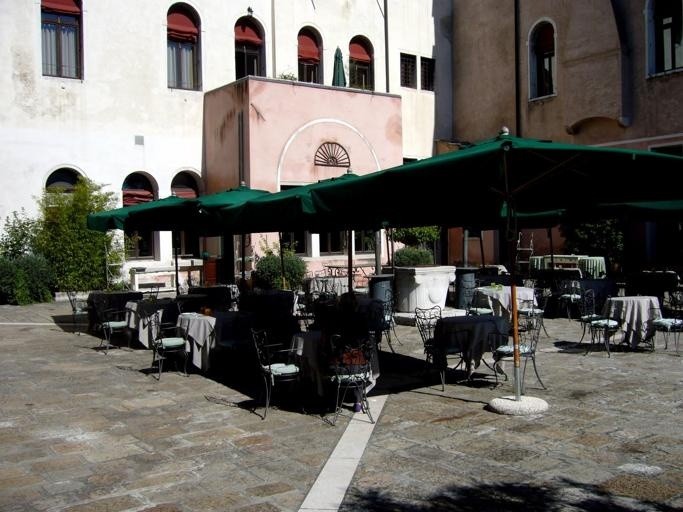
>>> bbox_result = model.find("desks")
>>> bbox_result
[64,256,663,424]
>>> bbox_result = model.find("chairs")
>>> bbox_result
[647,292,683,356]
